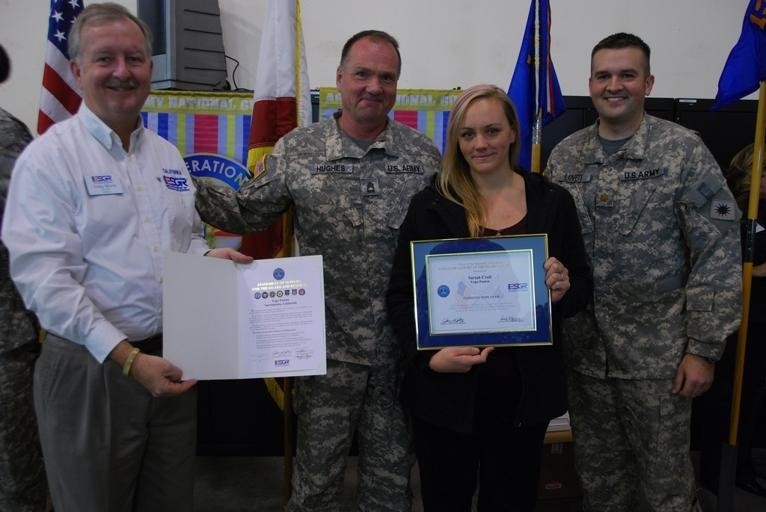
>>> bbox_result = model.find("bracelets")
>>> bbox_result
[122,346,140,378]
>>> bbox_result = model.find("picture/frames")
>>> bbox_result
[410,233,555,350]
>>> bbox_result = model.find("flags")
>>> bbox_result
[712,0,766,113]
[505,0,569,172]
[35,1,86,138]
[240,0,311,412]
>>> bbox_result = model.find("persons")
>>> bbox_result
[0,0,255,510]
[384,83,596,511]
[190,28,444,512]
[542,32,745,512]
